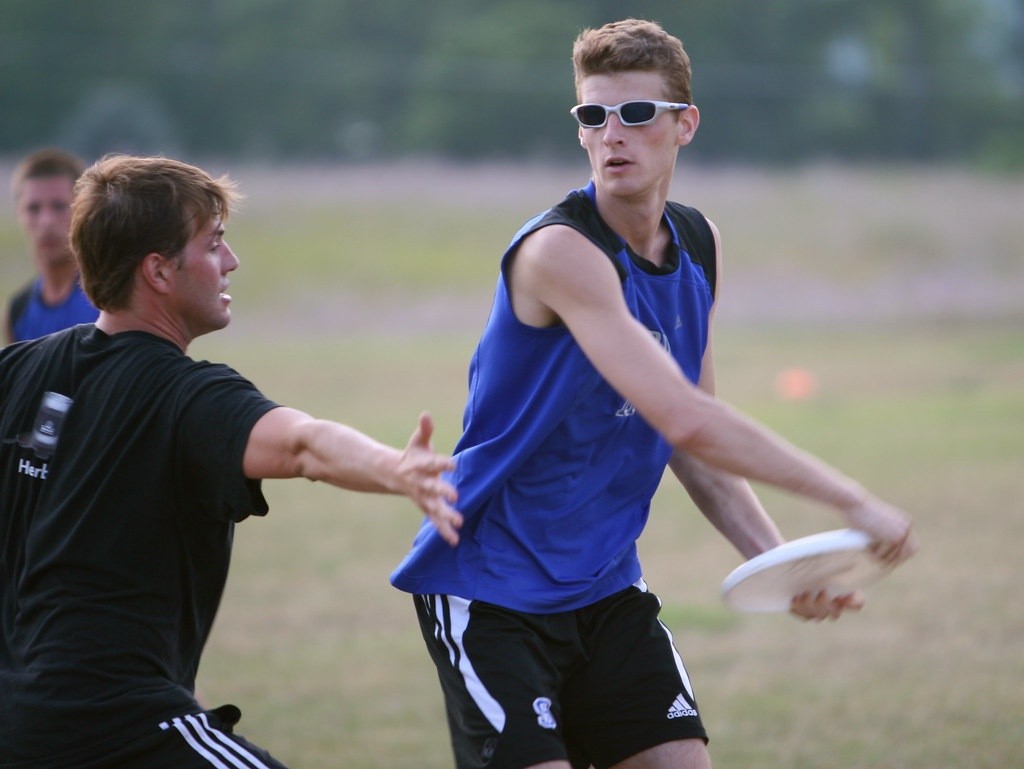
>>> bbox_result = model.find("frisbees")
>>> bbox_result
[719,527,898,618]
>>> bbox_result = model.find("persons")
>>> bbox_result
[0,151,101,346]
[0,156,463,769]
[391,21,913,769]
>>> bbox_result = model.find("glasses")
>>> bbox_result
[570,100,689,128]
